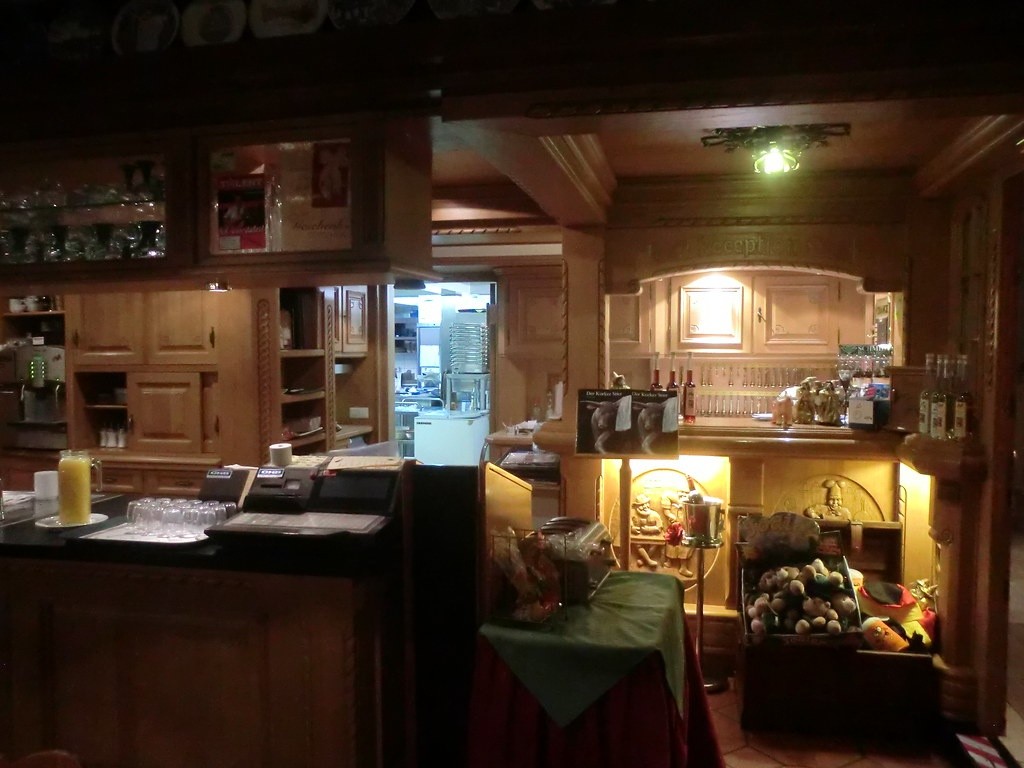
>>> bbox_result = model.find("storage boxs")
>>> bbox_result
[734,512,863,649]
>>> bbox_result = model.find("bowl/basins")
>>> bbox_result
[27,295,51,311]
[269,443,292,466]
[11,305,28,313]
[406,431,414,439]
[395,426,410,439]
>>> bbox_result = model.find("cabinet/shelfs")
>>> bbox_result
[0,111,443,498]
[669,271,843,359]
[503,273,659,357]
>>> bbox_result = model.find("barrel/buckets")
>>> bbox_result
[676,497,726,543]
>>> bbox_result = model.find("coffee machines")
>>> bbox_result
[14,346,65,424]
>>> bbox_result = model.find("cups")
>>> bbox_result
[9,299,22,312]
[126,497,236,539]
[33,471,58,500]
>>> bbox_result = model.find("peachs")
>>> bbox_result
[748,560,843,632]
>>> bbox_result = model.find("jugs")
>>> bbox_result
[58,448,102,524]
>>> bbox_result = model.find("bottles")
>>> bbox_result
[679,367,685,419]
[684,352,696,424]
[919,352,974,442]
[650,352,663,392]
[666,352,680,424]
[685,475,703,504]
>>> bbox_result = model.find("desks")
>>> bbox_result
[471,569,728,768]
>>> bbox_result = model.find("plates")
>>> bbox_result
[34,513,109,527]
